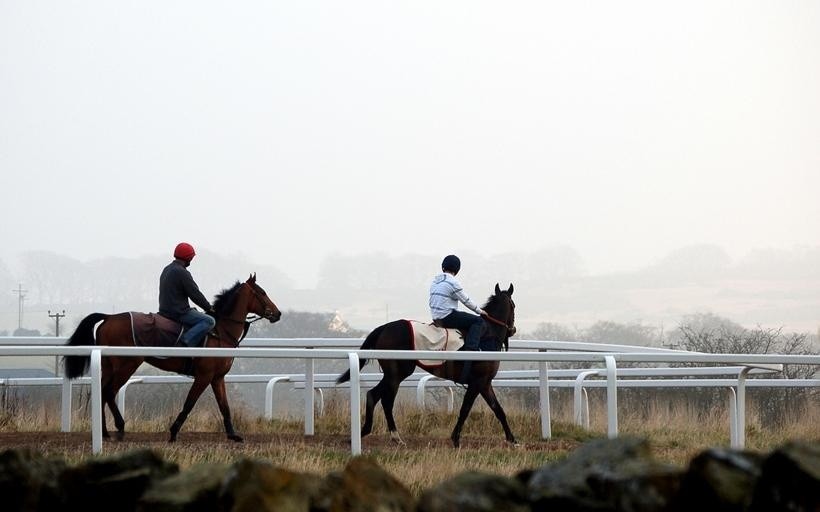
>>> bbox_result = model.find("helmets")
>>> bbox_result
[442,255,460,274]
[175,243,195,260]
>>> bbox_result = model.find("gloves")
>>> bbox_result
[209,306,216,313]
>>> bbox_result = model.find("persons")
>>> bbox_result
[431,255,488,350]
[161,243,216,344]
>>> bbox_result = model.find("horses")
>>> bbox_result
[335,282,521,449]
[60,272,282,444]
